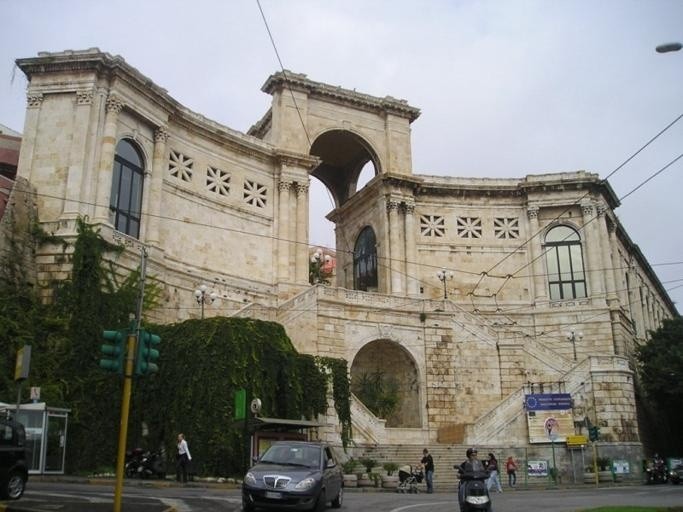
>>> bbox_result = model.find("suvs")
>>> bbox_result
[0,407,29,501]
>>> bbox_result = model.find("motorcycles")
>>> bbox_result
[453,458,495,511]
[643,463,669,485]
[125,448,167,479]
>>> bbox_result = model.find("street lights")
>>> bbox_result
[437,266,454,298]
[311,248,331,284]
[195,284,217,321]
[565,327,584,362]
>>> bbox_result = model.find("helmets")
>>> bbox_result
[466,448,478,458]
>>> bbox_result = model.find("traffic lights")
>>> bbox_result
[143,332,160,377]
[100,330,123,372]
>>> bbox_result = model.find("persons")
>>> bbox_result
[651,453,664,475]
[458,446,491,488]
[416,447,435,494]
[485,451,503,493]
[173,432,192,484]
[505,455,518,488]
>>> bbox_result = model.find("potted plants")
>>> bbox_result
[381,463,400,486]
[597,456,613,481]
[342,456,357,486]
[357,456,378,486]
[583,464,597,483]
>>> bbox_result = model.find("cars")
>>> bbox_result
[670,462,683,486]
[242,440,344,512]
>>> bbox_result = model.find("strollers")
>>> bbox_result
[396,463,424,494]
